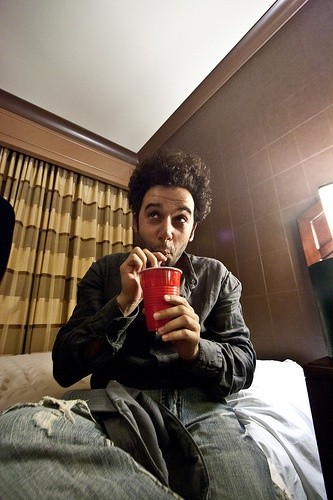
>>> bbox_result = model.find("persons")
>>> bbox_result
[0,152,289,500]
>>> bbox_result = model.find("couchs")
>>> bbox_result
[0,349,328,500]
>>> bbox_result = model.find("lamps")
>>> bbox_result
[318,182,333,238]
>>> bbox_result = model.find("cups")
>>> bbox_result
[140,267,183,331]
